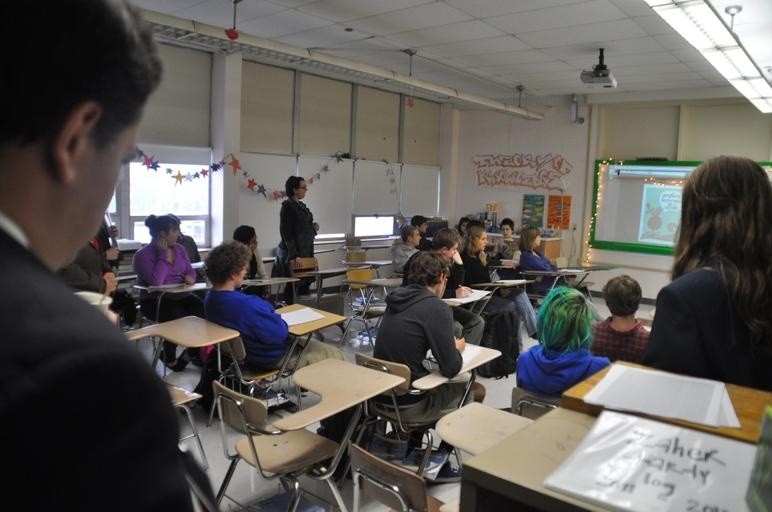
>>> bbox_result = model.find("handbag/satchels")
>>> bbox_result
[109,289,138,326]
[316,405,368,480]
[192,349,233,418]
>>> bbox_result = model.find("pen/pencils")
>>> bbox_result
[458,285,474,293]
[493,282,504,284]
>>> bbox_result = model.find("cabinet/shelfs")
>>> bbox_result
[561,360,772,444]
[484,230,560,259]
[459,408,772,512]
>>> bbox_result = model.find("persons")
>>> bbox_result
[133,213,207,372]
[372,248,488,488]
[516,285,613,398]
[591,274,651,365]
[390,214,566,367]
[280,175,318,305]
[54,219,121,296]
[199,225,351,376]
[639,155,772,393]
[2,1,193,511]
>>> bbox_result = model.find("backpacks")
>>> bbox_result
[477,311,520,378]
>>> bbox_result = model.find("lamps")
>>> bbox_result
[569,93,578,123]
[645,0,772,115]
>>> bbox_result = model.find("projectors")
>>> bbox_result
[580,68,618,91]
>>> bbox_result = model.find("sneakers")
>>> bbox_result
[159,350,190,371]
[425,460,462,483]
